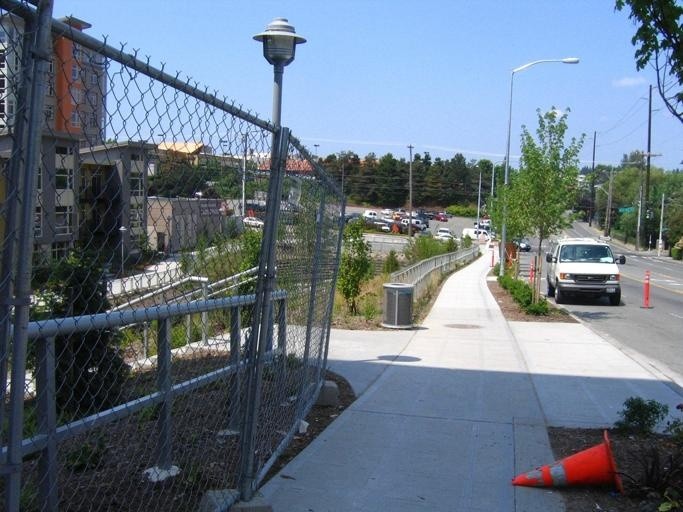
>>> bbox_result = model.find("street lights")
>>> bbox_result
[475,164,481,243]
[251,15,306,353]
[497,56,579,279]
[118,225,126,274]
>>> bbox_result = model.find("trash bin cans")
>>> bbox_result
[380,283,416,329]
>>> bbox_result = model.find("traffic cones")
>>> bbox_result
[511,430,624,495]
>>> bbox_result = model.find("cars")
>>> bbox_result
[337,209,495,243]
[544,238,625,306]
[511,239,530,252]
[242,216,264,228]
